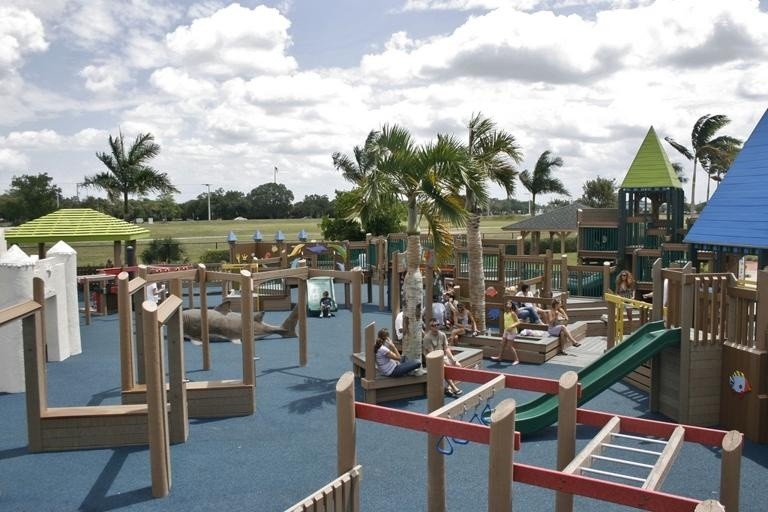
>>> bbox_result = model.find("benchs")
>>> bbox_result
[349,329,491,404]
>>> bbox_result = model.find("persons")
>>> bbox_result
[394,281,480,346]
[374,328,424,377]
[320,291,337,318]
[515,284,547,324]
[642,278,669,306]
[490,300,521,366]
[422,317,463,397]
[615,270,636,321]
[646,222,664,242]
[547,299,582,355]
[511,300,544,325]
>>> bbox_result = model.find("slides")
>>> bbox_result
[306,276,338,312]
[482,320,682,434]
[554,267,614,292]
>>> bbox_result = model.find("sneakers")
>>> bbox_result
[320,315,323,318]
[573,343,581,347]
[491,357,501,361]
[445,388,453,396]
[454,388,462,395]
[512,360,519,365]
[328,314,331,317]
[560,350,567,355]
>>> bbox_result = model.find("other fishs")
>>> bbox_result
[182,301,299,346]
